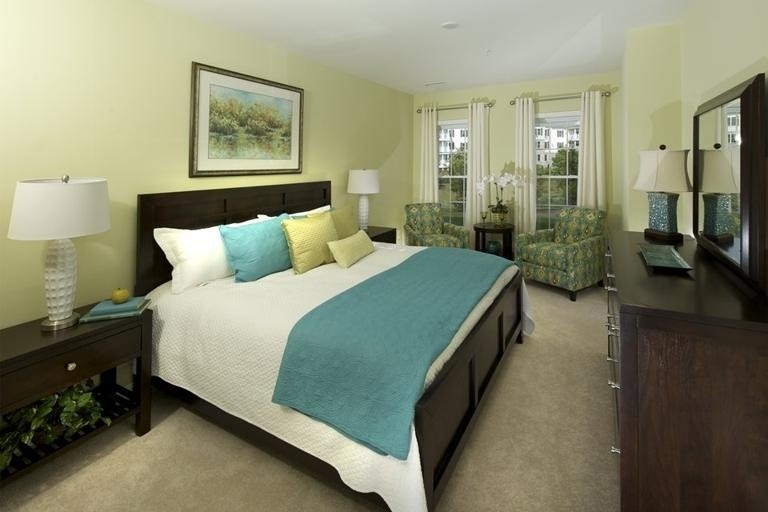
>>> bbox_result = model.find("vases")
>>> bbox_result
[489,203,509,225]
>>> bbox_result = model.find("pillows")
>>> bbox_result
[219,213,295,282]
[304,204,360,240]
[280,211,339,275]
[256,205,331,219]
[152,216,279,295]
[326,229,376,268]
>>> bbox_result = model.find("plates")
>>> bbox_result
[637,242,694,275]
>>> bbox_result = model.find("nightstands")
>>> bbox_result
[0,293,154,490]
[361,225,396,244]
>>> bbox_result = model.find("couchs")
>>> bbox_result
[514,206,606,301]
[402,202,470,249]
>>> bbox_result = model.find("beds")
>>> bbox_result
[134,179,525,511]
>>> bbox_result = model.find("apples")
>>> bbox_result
[112,287,130,303]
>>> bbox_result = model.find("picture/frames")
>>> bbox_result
[188,60,305,179]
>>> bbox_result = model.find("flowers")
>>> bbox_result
[477,173,523,212]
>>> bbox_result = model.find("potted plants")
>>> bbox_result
[0,378,112,473]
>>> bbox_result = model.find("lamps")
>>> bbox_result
[347,169,380,234]
[6,175,111,332]
[631,144,692,245]
[697,143,737,243]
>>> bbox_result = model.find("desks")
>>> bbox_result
[599,228,768,511]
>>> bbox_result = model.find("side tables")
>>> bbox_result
[472,221,515,262]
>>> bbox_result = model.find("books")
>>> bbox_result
[78,295,153,324]
[88,295,146,316]
